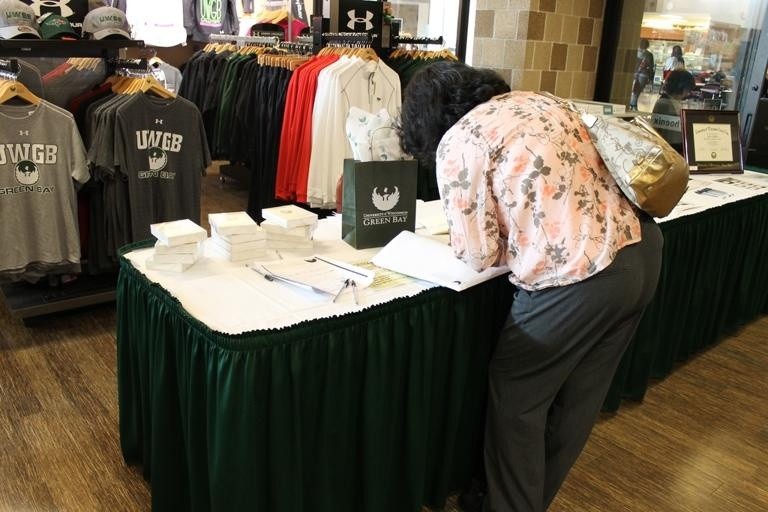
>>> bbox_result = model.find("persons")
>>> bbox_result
[394,60,666,512]
[628,40,655,111]
[660,43,686,85]
[650,69,696,144]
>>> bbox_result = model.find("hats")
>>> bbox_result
[41,14,80,40]
[0,0,42,40]
[80,5,132,40]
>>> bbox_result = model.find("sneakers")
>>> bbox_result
[458,492,483,512]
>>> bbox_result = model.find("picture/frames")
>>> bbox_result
[679,108,745,175]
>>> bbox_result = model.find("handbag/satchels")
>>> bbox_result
[581,108,690,218]
[341,156,419,249]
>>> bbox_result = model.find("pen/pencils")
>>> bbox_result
[350,281,360,305]
[330,279,350,303]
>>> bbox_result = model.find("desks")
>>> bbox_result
[114,166,765,508]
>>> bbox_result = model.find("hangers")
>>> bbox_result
[203,38,265,56]
[316,33,458,62]
[0,72,40,107]
[66,50,177,99]
